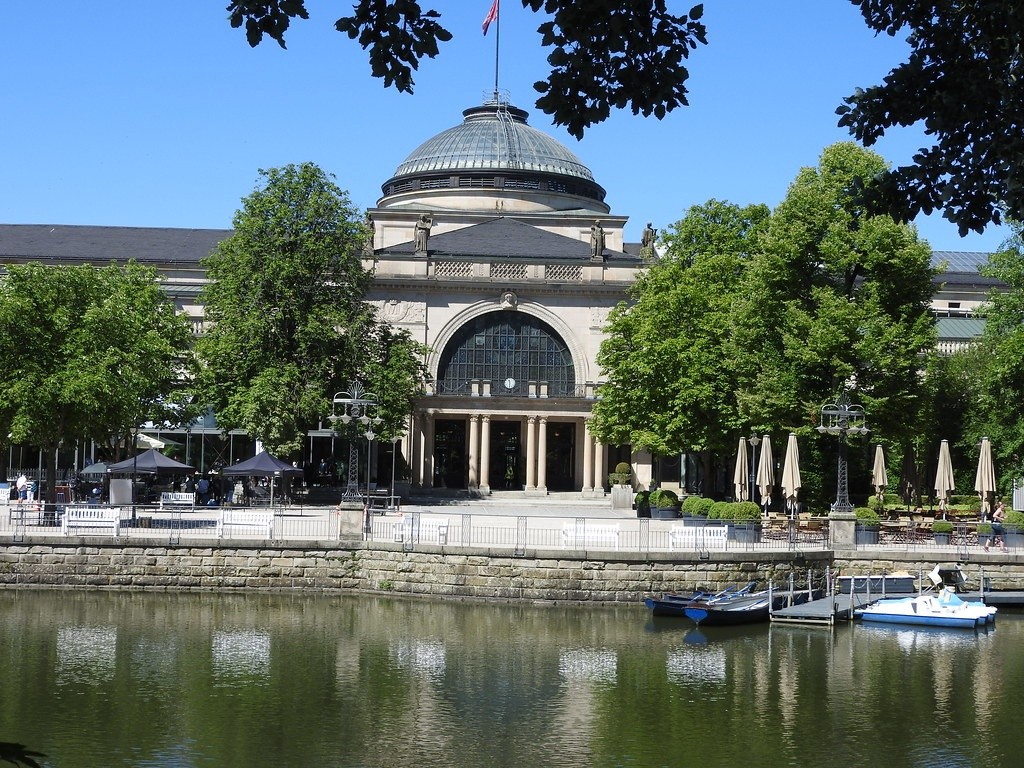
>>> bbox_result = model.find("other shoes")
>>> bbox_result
[1000,548,1008,552]
[982,546,990,553]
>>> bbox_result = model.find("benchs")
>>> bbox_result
[160,492,197,511]
[62,506,121,535]
[217,508,275,539]
[836,574,916,593]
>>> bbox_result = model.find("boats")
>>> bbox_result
[644,581,822,627]
[854,584,998,630]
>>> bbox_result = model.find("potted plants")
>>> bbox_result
[720,502,738,540]
[634,489,652,517]
[681,495,702,525]
[978,523,995,545]
[648,490,664,518]
[705,501,725,527]
[854,505,881,544]
[932,520,953,545]
[1004,510,1024,547]
[657,491,679,518]
[692,498,714,527]
[734,500,762,544]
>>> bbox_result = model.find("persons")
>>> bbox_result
[173,475,270,506]
[984,501,1009,553]
[16,473,35,507]
[318,459,327,475]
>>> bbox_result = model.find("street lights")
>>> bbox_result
[327,377,385,502]
[747,432,762,503]
[813,391,871,513]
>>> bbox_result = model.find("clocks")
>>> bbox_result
[504,377,516,389]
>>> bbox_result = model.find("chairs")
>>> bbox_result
[761,512,1000,546]
[922,563,976,594]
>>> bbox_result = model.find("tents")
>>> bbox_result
[221,450,303,503]
[105,447,196,481]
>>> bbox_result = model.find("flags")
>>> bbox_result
[482,0,498,35]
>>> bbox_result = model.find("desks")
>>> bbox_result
[937,569,962,590]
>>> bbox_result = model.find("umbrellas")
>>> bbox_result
[80,463,111,473]
[974,437,996,521]
[781,433,801,517]
[755,435,775,516]
[934,440,955,520]
[733,437,750,501]
[871,445,888,500]
